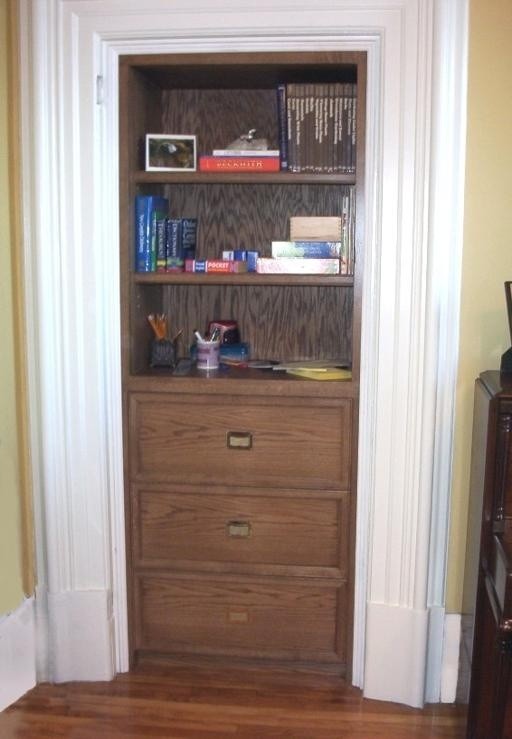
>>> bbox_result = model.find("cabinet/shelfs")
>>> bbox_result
[114,52,369,697]
[466,370,512,738]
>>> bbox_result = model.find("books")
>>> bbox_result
[134,185,356,276]
[272,359,352,381]
[198,146,284,172]
[279,81,357,175]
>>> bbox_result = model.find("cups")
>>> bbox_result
[196,339,221,369]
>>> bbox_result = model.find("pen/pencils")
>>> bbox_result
[147,312,167,340]
[174,329,183,341]
[194,328,220,341]
